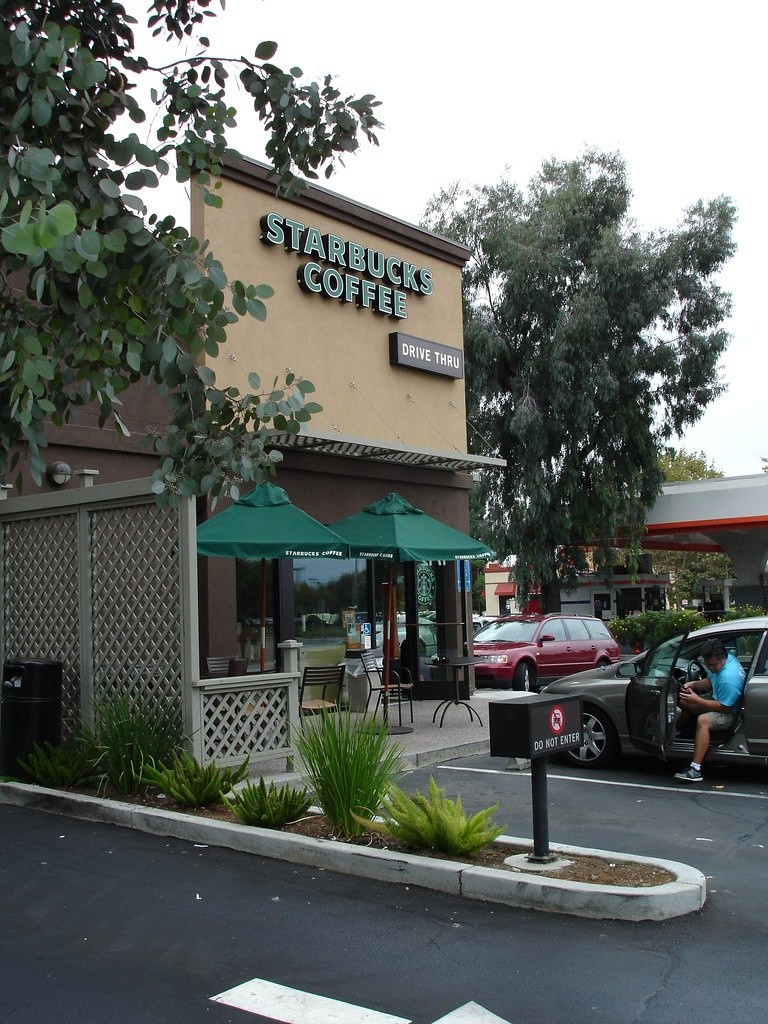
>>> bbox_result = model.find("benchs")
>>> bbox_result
[206,655,239,673]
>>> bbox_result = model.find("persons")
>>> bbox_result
[674,640,748,781]
[238,625,262,662]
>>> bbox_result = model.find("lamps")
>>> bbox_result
[46,461,72,486]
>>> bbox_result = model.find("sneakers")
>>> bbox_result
[674,766,703,781]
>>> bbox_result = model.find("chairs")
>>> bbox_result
[400,637,427,666]
[358,652,415,727]
[227,657,249,672]
[281,664,346,742]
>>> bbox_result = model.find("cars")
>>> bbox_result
[364,614,440,657]
[543,617,768,770]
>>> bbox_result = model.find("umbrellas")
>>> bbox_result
[326,491,496,729]
[196,479,351,673]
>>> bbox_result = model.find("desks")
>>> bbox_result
[423,657,487,728]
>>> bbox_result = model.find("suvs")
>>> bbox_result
[464,612,621,693]
